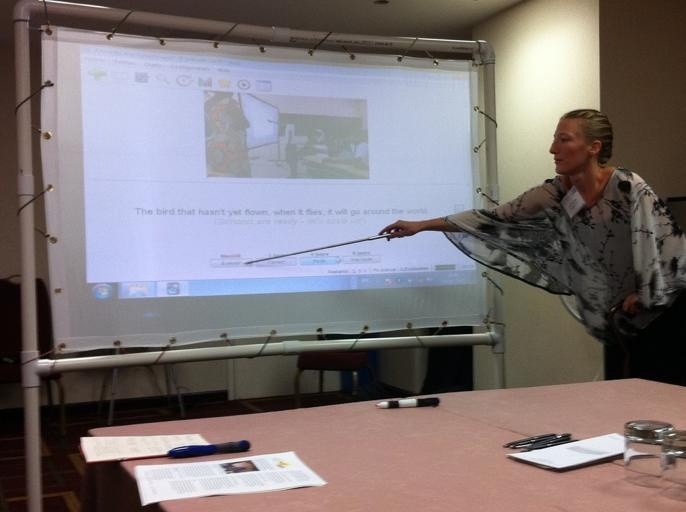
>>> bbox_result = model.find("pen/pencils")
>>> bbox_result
[375,398,439,409]
[169,440,250,458]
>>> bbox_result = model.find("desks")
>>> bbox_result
[87,377,686,512]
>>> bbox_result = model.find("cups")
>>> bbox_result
[623,421,672,487]
[660,430,686,501]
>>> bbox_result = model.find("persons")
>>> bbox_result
[378,109,686,389]
